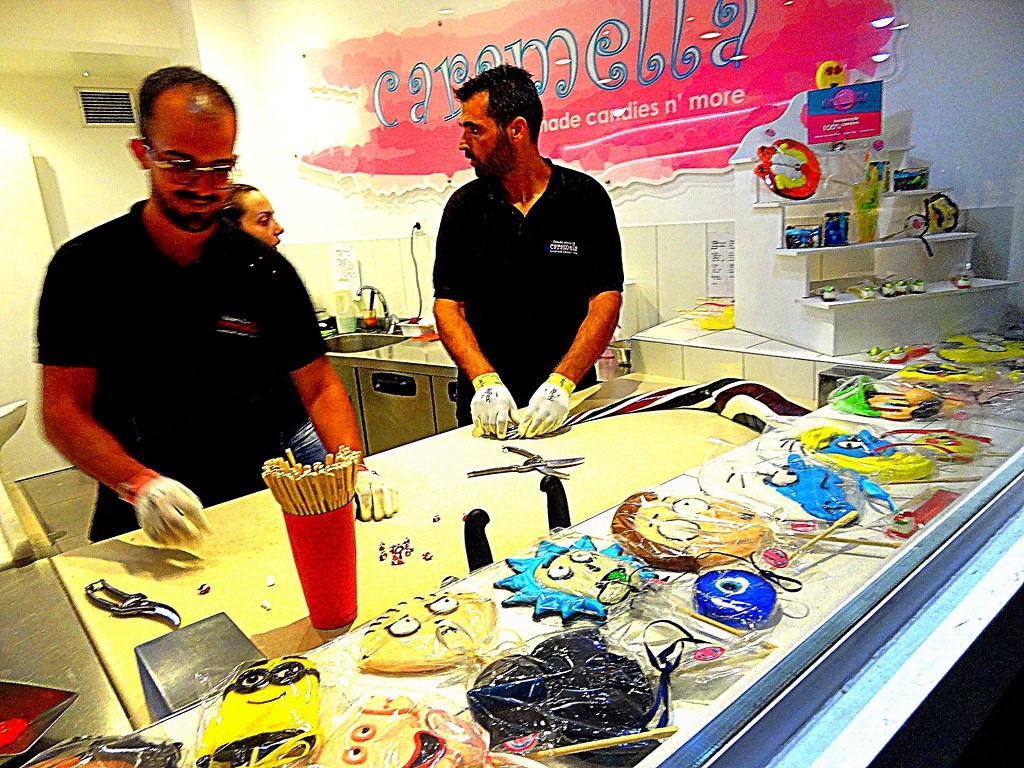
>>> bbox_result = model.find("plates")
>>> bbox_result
[609,339,631,367]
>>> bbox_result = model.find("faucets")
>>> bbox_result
[356,284,404,334]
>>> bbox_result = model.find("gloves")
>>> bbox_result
[351,464,399,521]
[121,470,214,549]
[470,373,520,439]
[517,373,575,439]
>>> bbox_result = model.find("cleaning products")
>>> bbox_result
[332,272,358,334]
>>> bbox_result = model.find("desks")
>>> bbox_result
[50,370,827,730]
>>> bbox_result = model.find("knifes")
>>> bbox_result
[85,581,183,630]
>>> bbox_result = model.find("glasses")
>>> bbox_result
[141,136,237,191]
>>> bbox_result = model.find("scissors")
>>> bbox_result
[85,579,181,627]
[467,445,586,478]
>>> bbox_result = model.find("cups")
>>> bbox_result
[281,500,358,629]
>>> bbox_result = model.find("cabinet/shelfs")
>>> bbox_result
[326,356,460,456]
[728,156,1018,356]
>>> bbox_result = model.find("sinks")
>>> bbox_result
[323,334,412,354]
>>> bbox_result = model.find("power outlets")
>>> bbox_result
[412,216,428,236]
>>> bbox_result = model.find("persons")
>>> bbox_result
[432,64,624,439]
[35,65,401,550]
